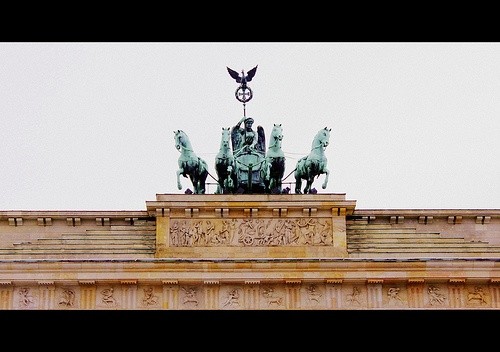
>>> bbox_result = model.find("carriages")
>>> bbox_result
[171,126,337,196]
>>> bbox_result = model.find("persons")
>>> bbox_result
[236,116,258,153]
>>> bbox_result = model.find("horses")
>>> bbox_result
[266,124,286,194]
[215,126,239,194]
[294,126,332,195]
[172,129,209,195]
